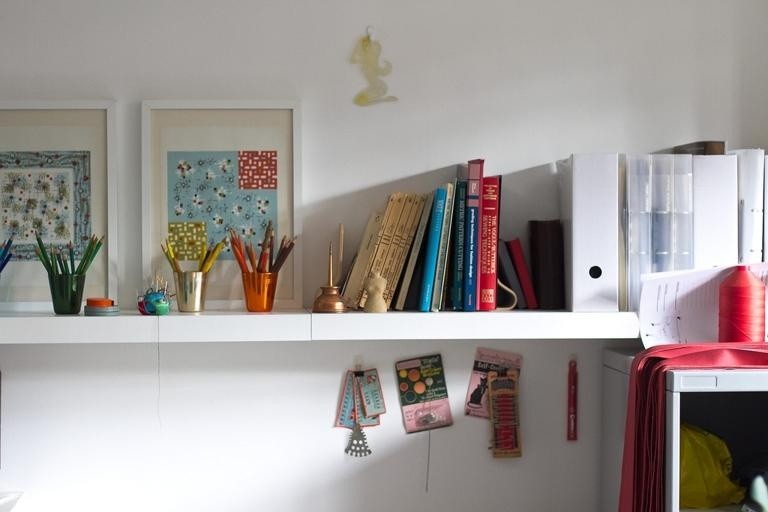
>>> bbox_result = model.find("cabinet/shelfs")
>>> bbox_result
[598,347,767,512]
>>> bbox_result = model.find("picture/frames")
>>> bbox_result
[0,100,119,314]
[141,99,304,311]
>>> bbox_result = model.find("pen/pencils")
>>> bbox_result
[231,220,298,271]
[0,233,15,273]
[160,236,225,271]
[32,229,105,274]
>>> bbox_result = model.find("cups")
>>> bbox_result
[173,271,208,312]
[241,271,279,312]
[48,273,86,314]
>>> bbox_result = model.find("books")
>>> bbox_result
[619,134,768,352]
[340,159,565,312]
